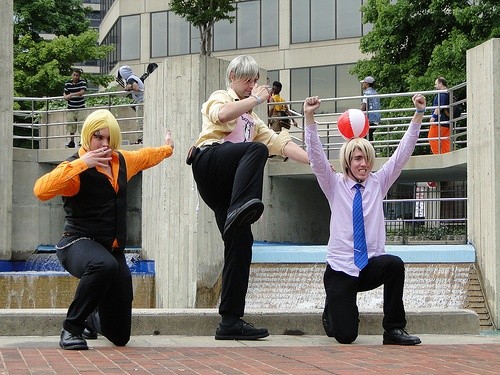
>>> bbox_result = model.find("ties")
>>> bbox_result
[353,183,369,270]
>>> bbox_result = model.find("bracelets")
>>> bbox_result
[432,113,438,119]
[416,109,426,113]
[251,93,262,104]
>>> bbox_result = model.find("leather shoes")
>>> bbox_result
[223,198,264,236]
[321,297,331,338]
[383,327,421,346]
[82,326,97,338]
[59,327,88,349]
[215,319,269,339]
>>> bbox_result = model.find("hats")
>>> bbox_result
[360,76,375,84]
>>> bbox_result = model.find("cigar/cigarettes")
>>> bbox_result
[101,149,104,152]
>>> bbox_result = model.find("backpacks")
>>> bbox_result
[444,94,463,118]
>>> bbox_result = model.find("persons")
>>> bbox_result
[117,61,158,145]
[427,76,453,155]
[268,80,298,163]
[302,92,427,346]
[359,75,383,158]
[63,69,87,151]
[186,54,336,347]
[32,108,176,350]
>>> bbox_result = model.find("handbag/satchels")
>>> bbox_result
[279,111,290,129]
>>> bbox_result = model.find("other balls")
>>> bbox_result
[337,108,369,141]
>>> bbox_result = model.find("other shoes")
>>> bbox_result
[133,140,143,145]
[65,141,75,148]
